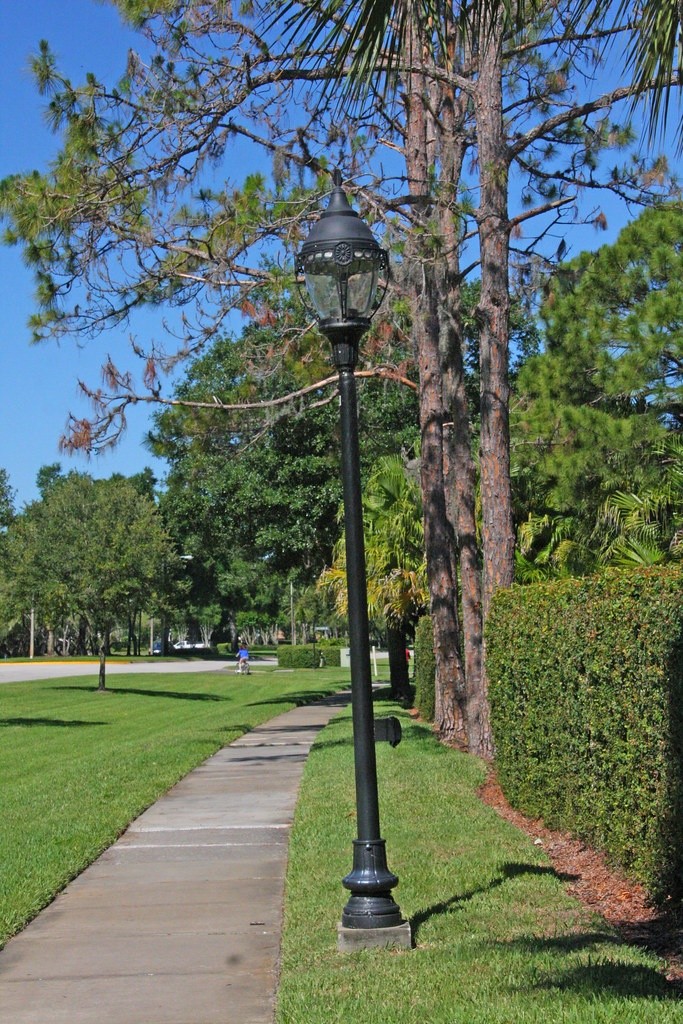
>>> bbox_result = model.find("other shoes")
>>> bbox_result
[237,670,241,673]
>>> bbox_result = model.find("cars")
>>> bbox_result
[149,639,175,656]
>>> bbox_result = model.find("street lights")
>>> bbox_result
[292,165,417,953]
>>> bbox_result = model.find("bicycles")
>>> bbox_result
[234,659,251,675]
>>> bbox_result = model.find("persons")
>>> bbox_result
[236,645,248,670]
[406,647,411,662]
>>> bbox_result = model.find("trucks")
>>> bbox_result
[171,640,208,651]
